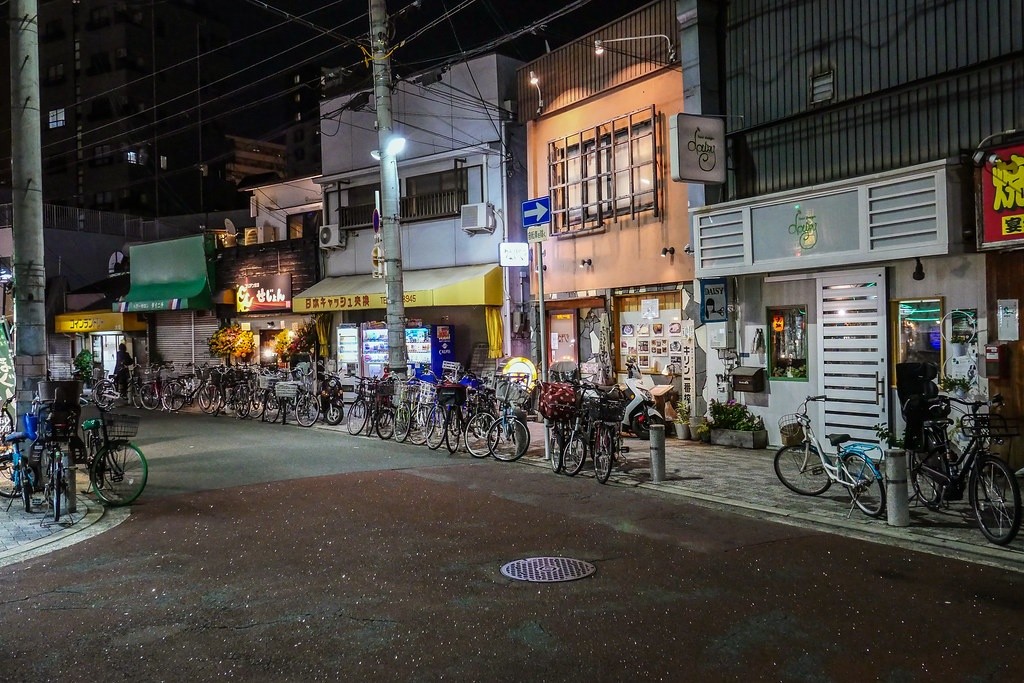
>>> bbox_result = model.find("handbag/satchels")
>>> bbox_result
[496,379,533,414]
[437,385,467,403]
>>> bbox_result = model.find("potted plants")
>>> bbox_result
[953,417,969,440]
[697,425,710,441]
[942,377,968,399]
[673,401,689,439]
[950,336,966,356]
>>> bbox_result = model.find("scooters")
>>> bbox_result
[620,361,666,441]
[315,360,347,425]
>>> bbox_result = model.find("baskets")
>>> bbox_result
[104,414,139,437]
[781,422,805,446]
[400,386,418,401]
[275,381,298,397]
[196,370,210,378]
[260,376,277,390]
[590,397,626,421]
[140,368,151,381]
[961,418,1021,436]
[376,381,393,396]
[355,381,372,396]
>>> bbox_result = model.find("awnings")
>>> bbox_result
[292,262,502,312]
[54,235,216,333]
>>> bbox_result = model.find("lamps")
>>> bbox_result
[580,259,591,268]
[912,259,925,281]
[594,34,675,59]
[660,247,674,257]
[530,71,544,108]
[535,264,546,272]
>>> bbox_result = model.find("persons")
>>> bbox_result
[895,352,927,451]
[115,344,134,397]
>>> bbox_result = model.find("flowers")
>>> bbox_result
[709,398,765,430]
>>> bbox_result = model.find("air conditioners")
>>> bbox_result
[460,203,493,229]
[319,224,345,247]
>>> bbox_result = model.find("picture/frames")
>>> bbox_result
[667,321,681,336]
[636,323,649,336]
[650,339,669,357]
[636,340,649,354]
[669,340,682,353]
[638,354,650,369]
[652,323,663,336]
[620,324,634,336]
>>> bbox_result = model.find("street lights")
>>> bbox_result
[369,137,407,407]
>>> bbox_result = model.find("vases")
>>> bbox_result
[711,428,767,449]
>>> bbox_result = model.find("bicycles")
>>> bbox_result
[0,394,41,512]
[343,371,499,459]
[532,370,627,484]
[28,397,87,527]
[74,398,149,506]
[773,395,886,519]
[91,361,320,427]
[487,373,530,462]
[905,395,1022,546]
